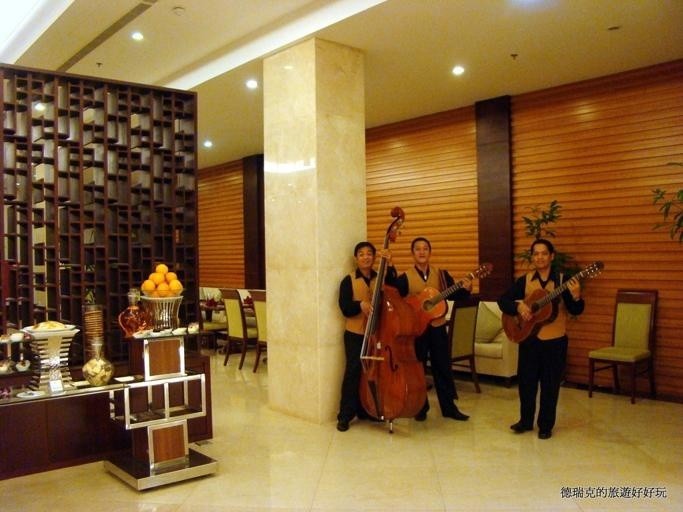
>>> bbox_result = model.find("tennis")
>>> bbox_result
[359,207,427,420]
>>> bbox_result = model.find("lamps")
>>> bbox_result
[426,294,484,394]
[199,286,267,373]
[585,285,657,403]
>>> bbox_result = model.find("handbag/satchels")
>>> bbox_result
[572,296,579,300]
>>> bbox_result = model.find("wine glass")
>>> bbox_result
[520,311,524,316]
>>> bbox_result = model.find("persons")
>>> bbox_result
[395,237,475,421]
[335,240,397,433]
[497,240,585,438]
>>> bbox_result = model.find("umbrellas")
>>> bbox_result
[415,405,429,421]
[336,419,349,432]
[510,422,532,432]
[443,408,470,421]
[539,430,551,439]
[358,416,385,422]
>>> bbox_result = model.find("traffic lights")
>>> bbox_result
[471,301,504,344]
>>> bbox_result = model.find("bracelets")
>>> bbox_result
[140,288,185,298]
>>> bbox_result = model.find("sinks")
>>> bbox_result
[425,301,520,390]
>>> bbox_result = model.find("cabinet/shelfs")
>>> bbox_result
[82,343,114,387]
[118,289,148,337]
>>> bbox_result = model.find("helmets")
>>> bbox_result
[17,390,46,401]
[23,323,77,333]
